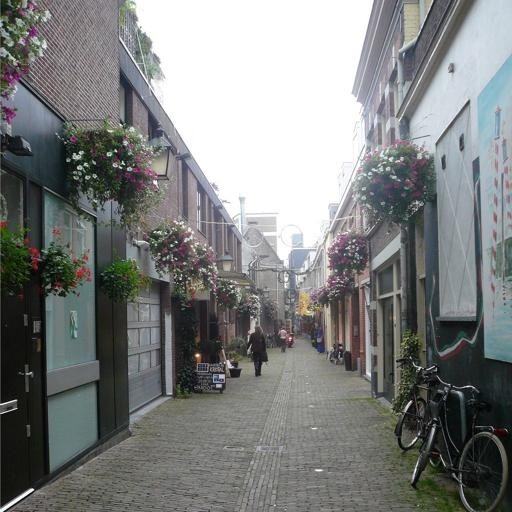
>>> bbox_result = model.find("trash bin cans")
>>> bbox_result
[344,351,352,371]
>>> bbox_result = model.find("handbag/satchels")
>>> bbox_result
[261,351,268,362]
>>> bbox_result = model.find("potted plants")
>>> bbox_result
[227,350,243,378]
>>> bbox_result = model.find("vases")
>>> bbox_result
[404,420,417,430]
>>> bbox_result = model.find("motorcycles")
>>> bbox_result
[287,333,294,347]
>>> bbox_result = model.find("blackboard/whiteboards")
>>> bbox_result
[195,362,226,391]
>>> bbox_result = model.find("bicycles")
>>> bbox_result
[328,342,343,364]
[389,356,510,512]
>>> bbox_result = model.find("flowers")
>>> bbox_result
[43,229,92,297]
[1,220,43,302]
[388,329,421,418]
[307,231,367,315]
[1,1,51,128]
[351,138,437,229]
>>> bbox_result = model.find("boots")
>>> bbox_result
[254,367,261,377]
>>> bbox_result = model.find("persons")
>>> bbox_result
[277,326,288,352]
[315,322,325,353]
[310,324,317,347]
[246,325,268,377]
